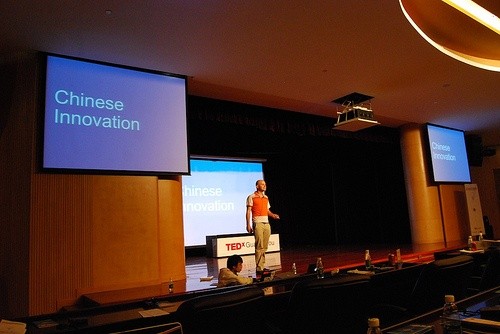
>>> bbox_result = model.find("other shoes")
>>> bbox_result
[256,268,271,275]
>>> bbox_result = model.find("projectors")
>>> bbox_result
[339,109,374,122]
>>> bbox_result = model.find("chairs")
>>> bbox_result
[175,244,500,334]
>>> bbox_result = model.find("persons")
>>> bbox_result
[245,180,280,275]
[217,254,253,288]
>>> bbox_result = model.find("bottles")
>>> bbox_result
[443,295,458,334]
[365,250,371,270]
[367,318,382,334]
[479,231,483,241]
[317,258,324,279]
[468,236,473,250]
[292,263,296,275]
[168,277,174,293]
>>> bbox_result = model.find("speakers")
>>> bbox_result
[464,134,483,167]
